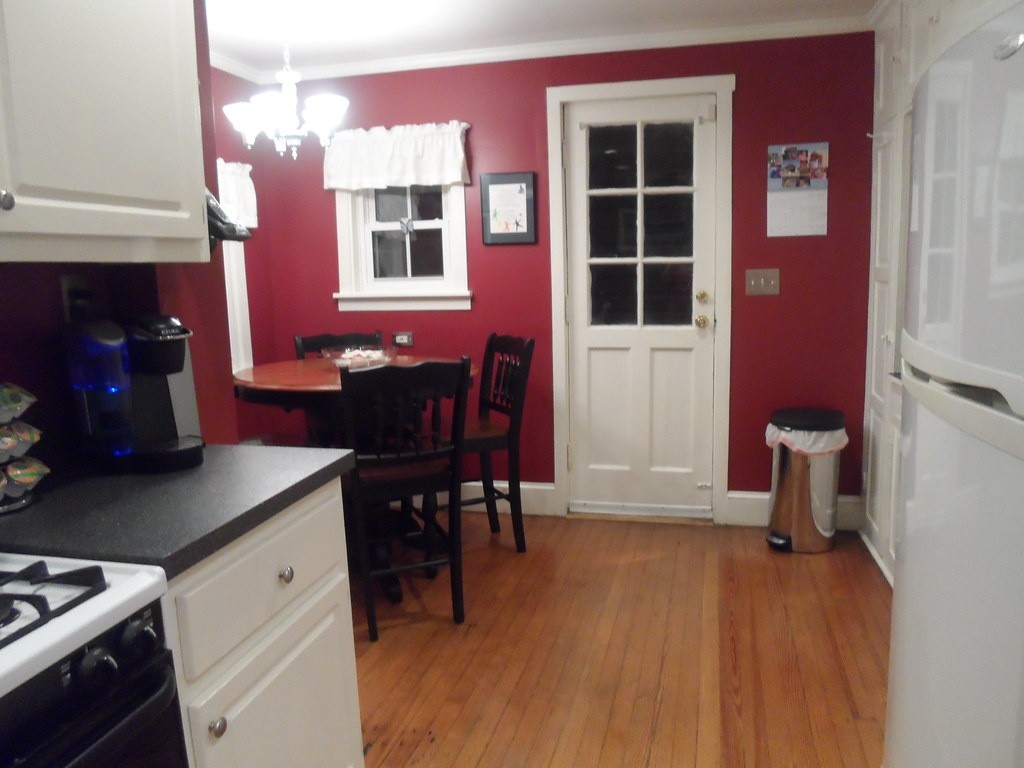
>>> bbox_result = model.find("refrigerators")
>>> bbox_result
[877,3,1023,768]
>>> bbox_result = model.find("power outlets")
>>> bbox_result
[393,332,413,348]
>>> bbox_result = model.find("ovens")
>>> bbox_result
[5,648,189,768]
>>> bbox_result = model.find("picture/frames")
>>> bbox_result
[481,172,537,245]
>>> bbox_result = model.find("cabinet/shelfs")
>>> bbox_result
[163,474,369,768]
[0,0,208,267]
[908,1,1023,82]
[864,0,912,584]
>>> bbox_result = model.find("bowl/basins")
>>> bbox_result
[320,343,398,371]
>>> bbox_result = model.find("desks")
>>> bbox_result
[234,355,478,604]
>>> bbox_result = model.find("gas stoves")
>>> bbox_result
[0,551,168,759]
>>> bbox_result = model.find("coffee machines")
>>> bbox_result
[54,285,206,470]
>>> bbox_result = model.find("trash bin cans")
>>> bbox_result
[762,408,846,553]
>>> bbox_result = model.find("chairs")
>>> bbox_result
[291,330,537,643]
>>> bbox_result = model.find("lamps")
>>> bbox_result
[222,46,349,159]
